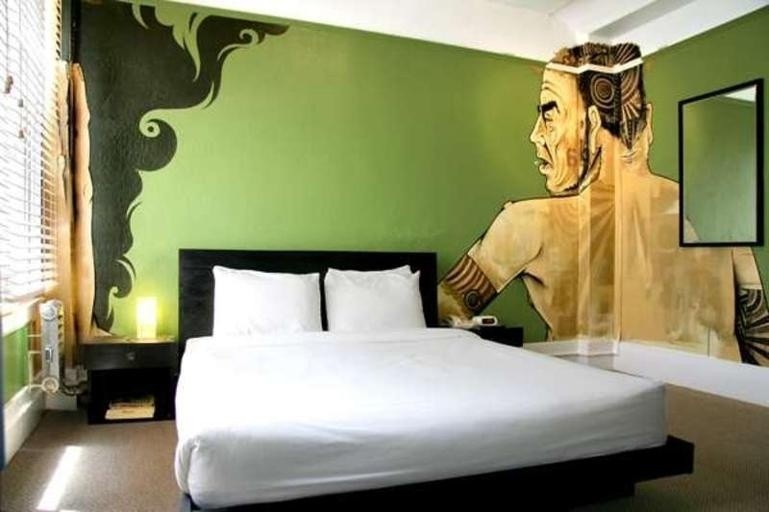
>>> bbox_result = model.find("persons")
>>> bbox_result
[437,37,768,368]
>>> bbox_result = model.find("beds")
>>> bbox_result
[173,248,695,512]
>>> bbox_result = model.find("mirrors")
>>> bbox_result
[678,78,764,247]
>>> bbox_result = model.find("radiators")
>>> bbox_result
[26,300,65,396]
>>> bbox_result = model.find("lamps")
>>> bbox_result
[135,298,157,340]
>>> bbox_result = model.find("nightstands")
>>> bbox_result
[82,336,177,424]
[439,325,524,348]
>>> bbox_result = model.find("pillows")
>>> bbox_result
[212,264,427,336]
